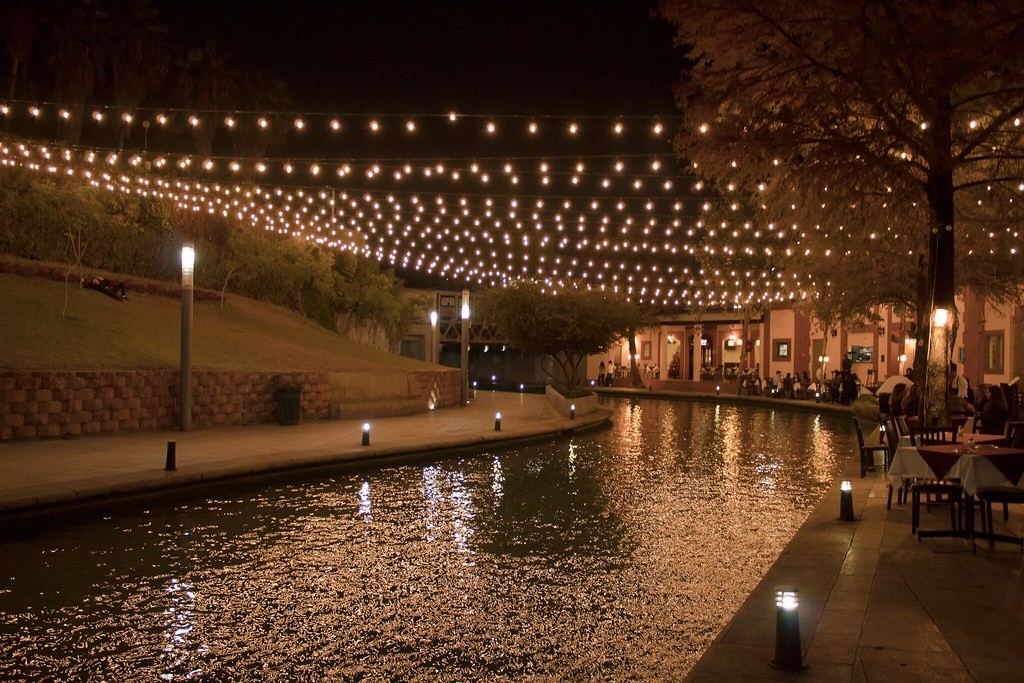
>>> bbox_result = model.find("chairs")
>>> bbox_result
[765,377,860,402]
[851,382,1024,549]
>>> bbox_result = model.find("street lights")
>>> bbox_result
[430,310,438,364]
[460,287,469,404]
[178,241,197,432]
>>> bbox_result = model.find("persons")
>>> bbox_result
[876,367,914,431]
[598,361,617,386]
[773,371,812,400]
[949,363,1007,435]
[829,354,858,404]
[637,363,659,379]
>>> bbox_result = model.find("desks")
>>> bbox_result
[895,431,1012,512]
[887,444,1024,556]
[908,413,972,433]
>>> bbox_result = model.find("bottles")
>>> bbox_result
[956,425,965,453]
[967,436,976,454]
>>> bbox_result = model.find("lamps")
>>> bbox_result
[496,413,501,419]
[591,381,594,385]
[772,388,774,393]
[841,481,852,491]
[363,423,369,431]
[880,425,885,432]
[774,588,799,610]
[717,385,719,390]
[815,393,819,397]
[571,404,575,410]
